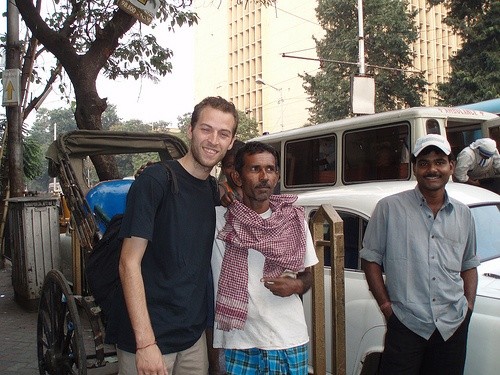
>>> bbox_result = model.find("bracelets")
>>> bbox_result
[137,343,156,350]
[379,302,391,312]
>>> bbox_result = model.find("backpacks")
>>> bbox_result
[90,160,217,344]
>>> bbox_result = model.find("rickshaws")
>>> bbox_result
[37,128,189,375]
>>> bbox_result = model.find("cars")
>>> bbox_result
[290,180,500,375]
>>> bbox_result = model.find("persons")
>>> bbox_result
[217,139,244,207]
[453,138,500,186]
[359,134,480,375]
[105,96,238,375]
[134,142,319,375]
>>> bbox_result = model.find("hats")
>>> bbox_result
[411,134,451,157]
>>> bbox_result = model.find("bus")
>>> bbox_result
[242,105,500,204]
